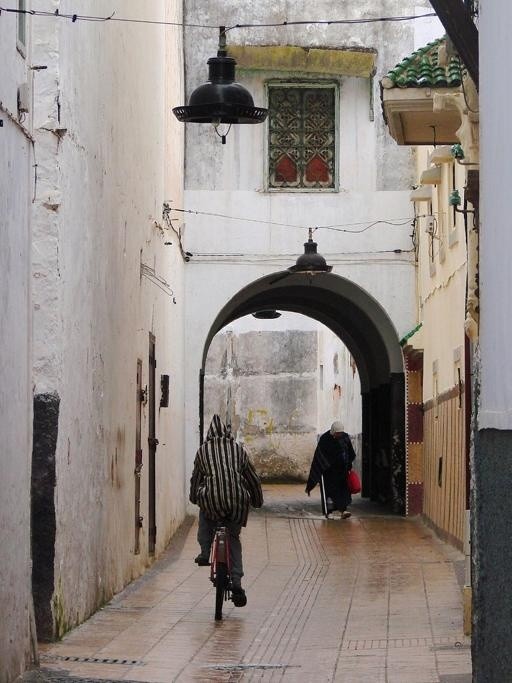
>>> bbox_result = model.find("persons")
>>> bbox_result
[305,421,356,521]
[189,413,264,608]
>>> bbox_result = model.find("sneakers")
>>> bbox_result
[232,585,246,607]
[327,514,334,520]
[341,511,351,519]
[195,554,209,564]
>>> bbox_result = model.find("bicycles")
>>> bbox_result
[196,518,245,620]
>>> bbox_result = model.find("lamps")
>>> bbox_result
[253,309,281,319]
[451,143,479,165]
[286,227,333,289]
[172,26,268,144]
[449,190,476,213]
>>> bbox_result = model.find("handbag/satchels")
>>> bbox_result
[347,469,361,494]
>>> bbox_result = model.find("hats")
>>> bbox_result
[330,421,345,435]
